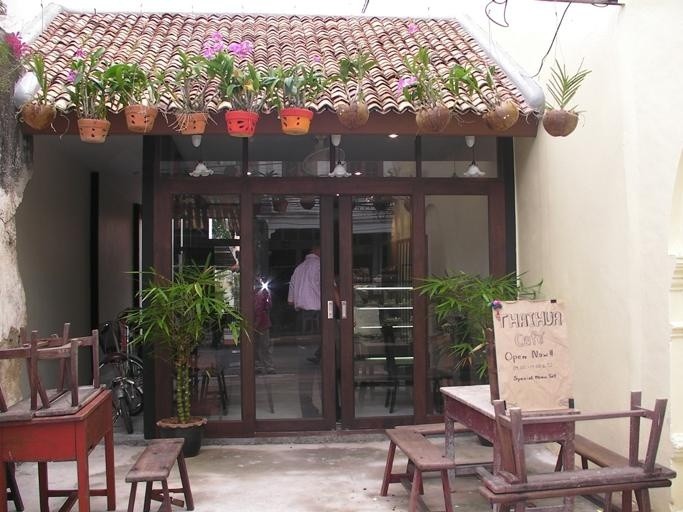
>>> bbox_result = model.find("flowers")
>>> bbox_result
[203,33,224,59]
[228,41,255,60]
[396,76,419,95]
[408,23,422,35]
[4,30,28,59]
[68,48,86,82]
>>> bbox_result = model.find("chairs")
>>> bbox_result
[183,307,412,417]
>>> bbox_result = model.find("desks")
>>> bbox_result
[438,382,582,512]
[0,388,115,512]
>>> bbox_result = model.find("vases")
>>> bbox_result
[75,117,111,143]
[175,112,209,137]
[19,105,57,129]
[224,110,260,137]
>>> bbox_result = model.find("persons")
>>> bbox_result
[255,273,276,374]
[287,241,345,423]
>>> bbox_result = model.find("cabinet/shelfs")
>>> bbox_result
[351,281,415,388]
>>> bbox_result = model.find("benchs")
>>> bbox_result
[553,432,629,511]
[394,420,474,482]
[379,426,456,511]
[18,325,107,419]
[124,436,195,511]
[0,321,70,424]
[482,390,677,495]
[477,478,671,511]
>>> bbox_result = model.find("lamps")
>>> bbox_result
[185,134,215,178]
[324,133,352,179]
[461,135,487,179]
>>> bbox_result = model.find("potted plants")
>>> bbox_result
[540,57,597,137]
[259,64,327,135]
[324,45,380,128]
[166,157,426,216]
[410,266,547,447]
[114,249,266,458]
[439,57,519,134]
[102,63,159,131]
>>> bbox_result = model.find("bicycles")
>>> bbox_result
[89,307,145,435]
